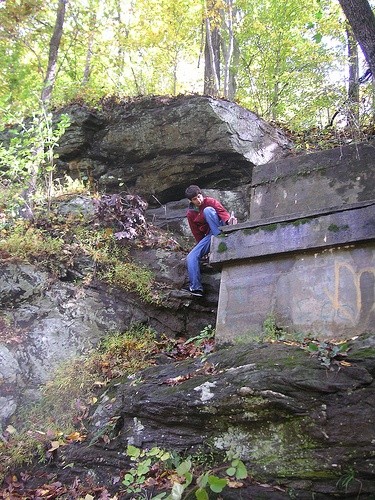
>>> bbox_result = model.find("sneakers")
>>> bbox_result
[181,287,203,296]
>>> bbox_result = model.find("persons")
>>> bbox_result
[185,185,236,296]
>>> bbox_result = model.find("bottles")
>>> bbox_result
[230,211,236,225]
